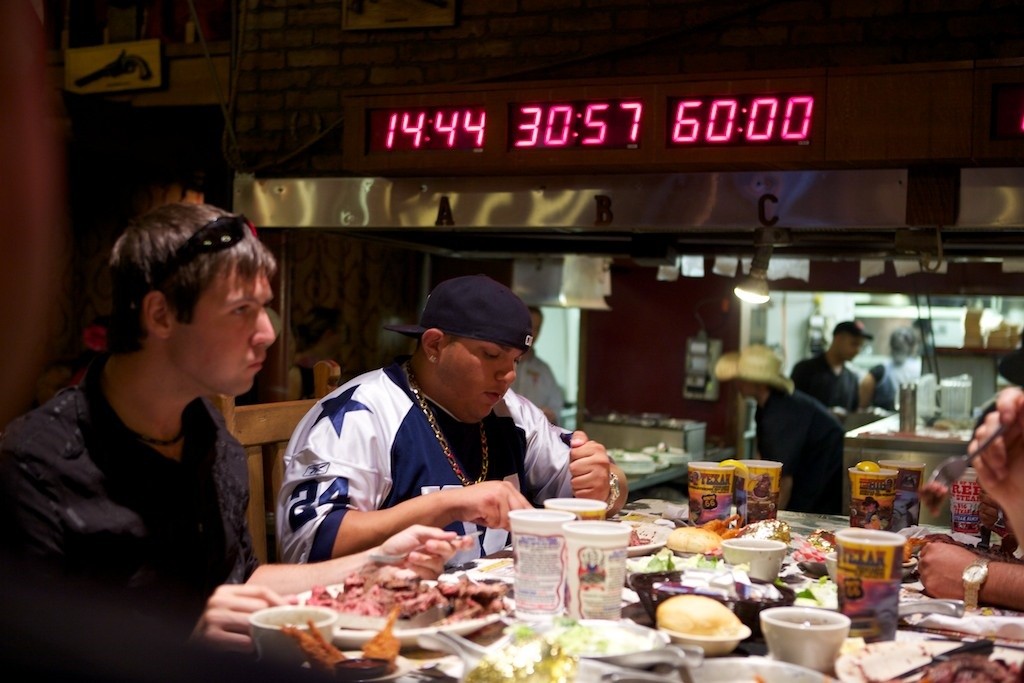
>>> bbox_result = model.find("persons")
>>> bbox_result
[275,275,629,566]
[0,202,477,683]
[715,319,933,514]
[509,306,562,424]
[919,388,1024,610]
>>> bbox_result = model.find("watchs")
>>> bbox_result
[605,473,620,513]
[961,556,990,608]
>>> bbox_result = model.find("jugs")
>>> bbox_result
[932,379,972,418]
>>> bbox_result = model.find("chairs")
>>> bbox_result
[207,359,340,563]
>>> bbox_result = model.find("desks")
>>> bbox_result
[387,497,1023,683]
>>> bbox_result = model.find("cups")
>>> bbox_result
[848,467,898,531]
[878,460,926,533]
[949,467,982,538]
[247,605,338,674]
[834,528,905,642]
[720,538,787,584]
[759,606,851,673]
[733,459,784,528]
[543,497,608,521]
[561,520,632,620]
[604,575,608,579]
[825,552,837,584]
[507,508,577,621]
[687,462,736,529]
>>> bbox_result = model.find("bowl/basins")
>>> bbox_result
[627,570,796,656]
[665,657,842,683]
[902,557,917,582]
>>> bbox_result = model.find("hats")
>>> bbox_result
[383,272,534,351]
[714,342,795,395]
[833,321,874,341]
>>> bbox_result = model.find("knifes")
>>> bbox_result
[889,639,995,683]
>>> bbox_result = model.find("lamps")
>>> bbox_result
[734,229,781,303]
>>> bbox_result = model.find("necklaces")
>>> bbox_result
[404,360,489,486]
[134,427,185,445]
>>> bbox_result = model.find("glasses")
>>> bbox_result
[147,213,258,291]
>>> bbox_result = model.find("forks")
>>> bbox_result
[922,423,1006,511]
[367,531,483,563]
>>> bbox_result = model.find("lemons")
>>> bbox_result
[717,459,749,479]
[855,460,880,472]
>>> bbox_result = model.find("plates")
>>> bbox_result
[300,580,517,649]
[794,569,838,612]
[620,521,667,558]
[608,446,691,474]
[834,639,1024,683]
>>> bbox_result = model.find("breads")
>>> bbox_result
[666,526,723,554]
[655,594,743,641]
